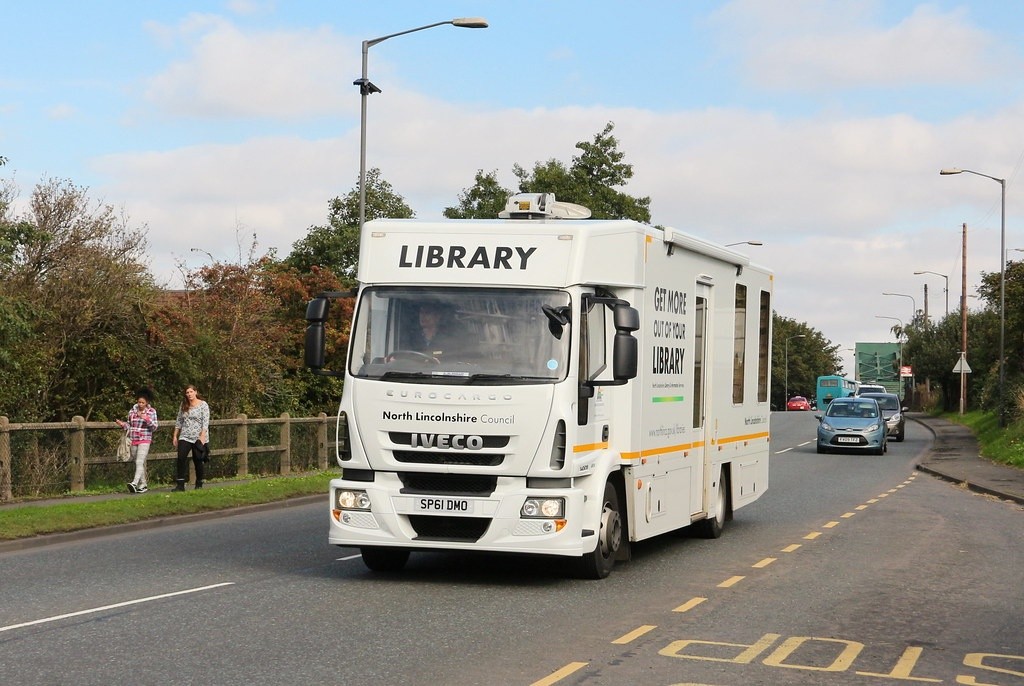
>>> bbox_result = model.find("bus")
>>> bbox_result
[815,376,857,412]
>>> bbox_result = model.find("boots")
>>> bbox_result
[194,480,204,489]
[171,479,185,492]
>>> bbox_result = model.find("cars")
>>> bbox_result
[814,397,888,455]
[787,396,811,411]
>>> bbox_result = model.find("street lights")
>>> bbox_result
[835,348,854,375]
[875,315,903,400]
[940,168,1009,429]
[913,270,949,319]
[1005,247,1024,267]
[883,292,915,324]
[357,16,489,234]
[785,334,806,411]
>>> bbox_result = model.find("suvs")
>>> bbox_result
[859,392,910,441]
[853,383,888,403]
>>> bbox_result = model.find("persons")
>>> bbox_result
[383,300,449,366]
[115,394,158,493]
[171,384,210,493]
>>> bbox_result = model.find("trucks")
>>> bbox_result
[305,185,779,584]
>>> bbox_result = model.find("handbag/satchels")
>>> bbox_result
[115,427,132,462]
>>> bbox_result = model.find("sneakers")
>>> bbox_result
[137,486,148,492]
[127,483,137,494]
